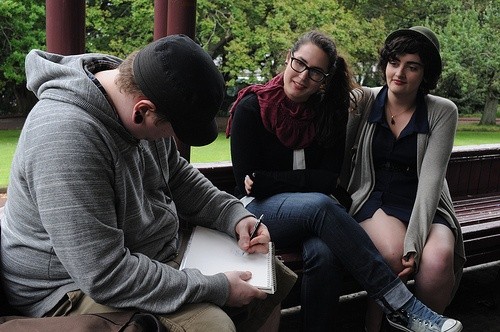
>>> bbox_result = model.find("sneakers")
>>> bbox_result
[386,294,462,332]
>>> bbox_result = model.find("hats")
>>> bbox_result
[382,25,443,80]
[132,34,226,148]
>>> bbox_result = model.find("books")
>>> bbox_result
[179,225,278,294]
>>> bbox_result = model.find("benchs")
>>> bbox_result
[191,143,500,319]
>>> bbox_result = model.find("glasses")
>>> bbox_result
[290,50,331,84]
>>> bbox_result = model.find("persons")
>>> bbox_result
[227,31,463,332]
[346,26,468,332]
[0,33,298,332]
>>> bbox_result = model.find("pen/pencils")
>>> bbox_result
[242,214,264,255]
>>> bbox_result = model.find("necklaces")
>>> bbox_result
[387,103,413,125]
